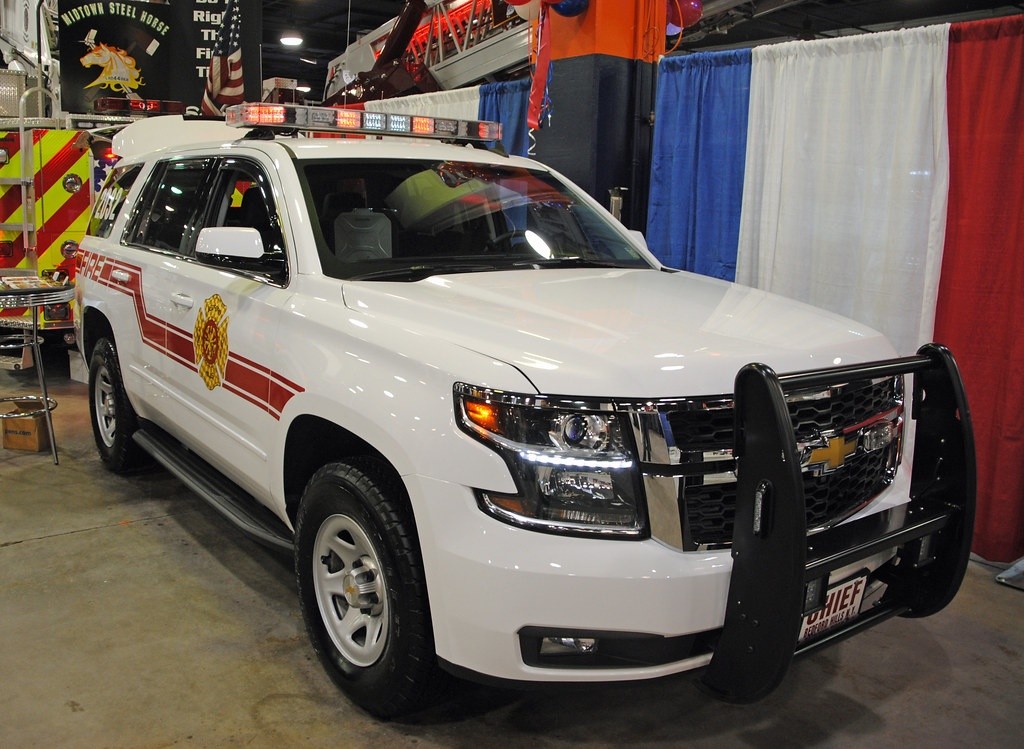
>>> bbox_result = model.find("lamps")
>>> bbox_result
[280,25,304,45]
[296,78,311,91]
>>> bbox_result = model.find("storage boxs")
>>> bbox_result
[2,396,53,451]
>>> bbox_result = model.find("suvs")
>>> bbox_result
[72,102,979,719]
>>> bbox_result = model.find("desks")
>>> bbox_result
[0,275,76,465]
